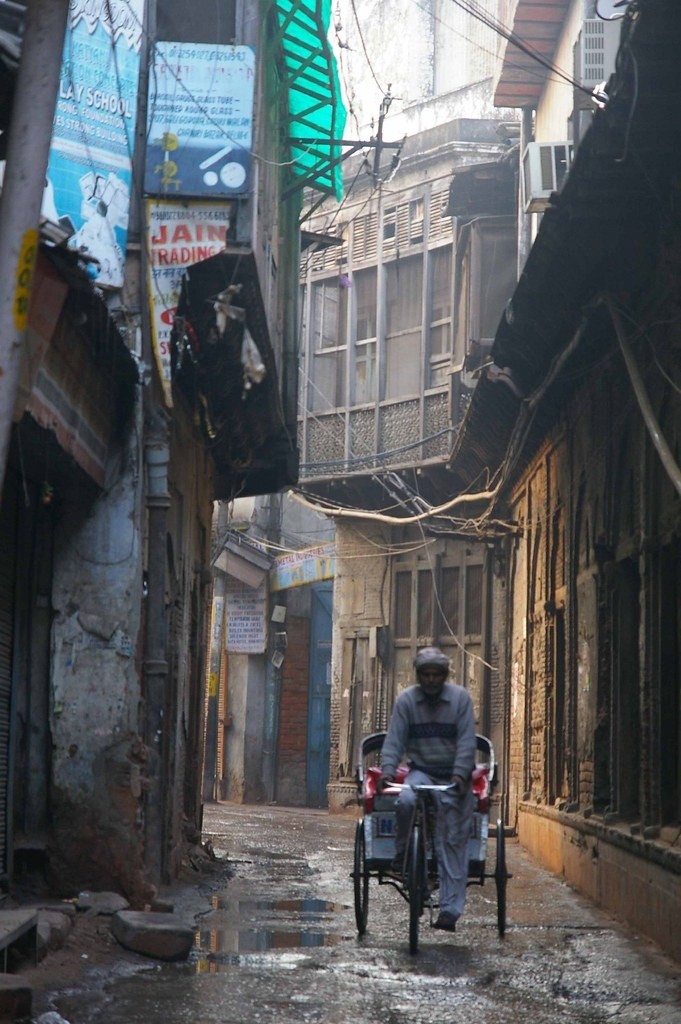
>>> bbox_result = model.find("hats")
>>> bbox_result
[413,646,449,670]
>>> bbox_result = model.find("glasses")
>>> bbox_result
[421,671,444,677]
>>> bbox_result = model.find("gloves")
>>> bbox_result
[374,774,394,795]
[450,775,467,796]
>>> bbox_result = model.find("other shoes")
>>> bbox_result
[391,853,408,872]
[434,911,457,932]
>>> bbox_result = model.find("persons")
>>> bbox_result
[381,653,477,930]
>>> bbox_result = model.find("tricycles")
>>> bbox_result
[349,732,517,955]
